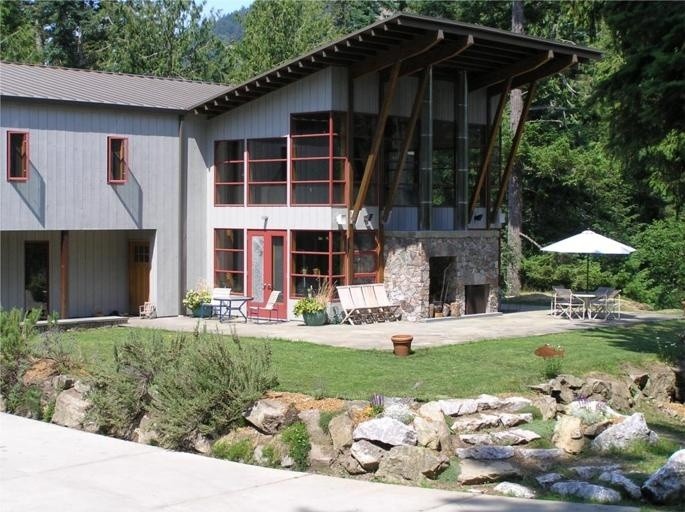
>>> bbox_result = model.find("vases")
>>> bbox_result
[391,334,414,357]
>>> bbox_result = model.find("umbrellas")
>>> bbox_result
[539,226,640,293]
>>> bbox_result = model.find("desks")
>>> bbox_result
[211,296,255,323]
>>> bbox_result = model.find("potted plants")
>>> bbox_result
[291,296,329,327]
[180,288,215,318]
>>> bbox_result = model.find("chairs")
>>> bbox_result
[249,290,281,324]
[549,282,622,321]
[200,286,231,321]
[332,282,401,325]
